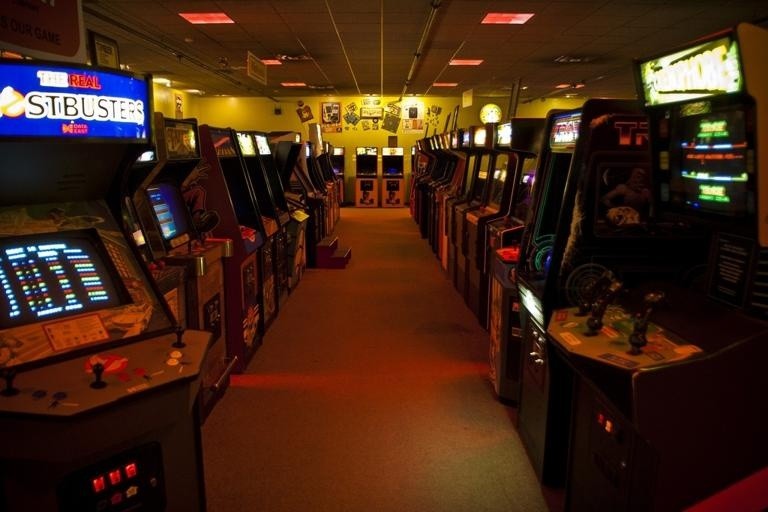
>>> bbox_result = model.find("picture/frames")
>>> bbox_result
[86,25,121,69]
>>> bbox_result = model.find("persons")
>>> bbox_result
[603,168,653,219]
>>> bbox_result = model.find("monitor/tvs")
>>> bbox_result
[464,155,541,228]
[0,228,126,331]
[665,104,750,219]
[149,185,183,242]
